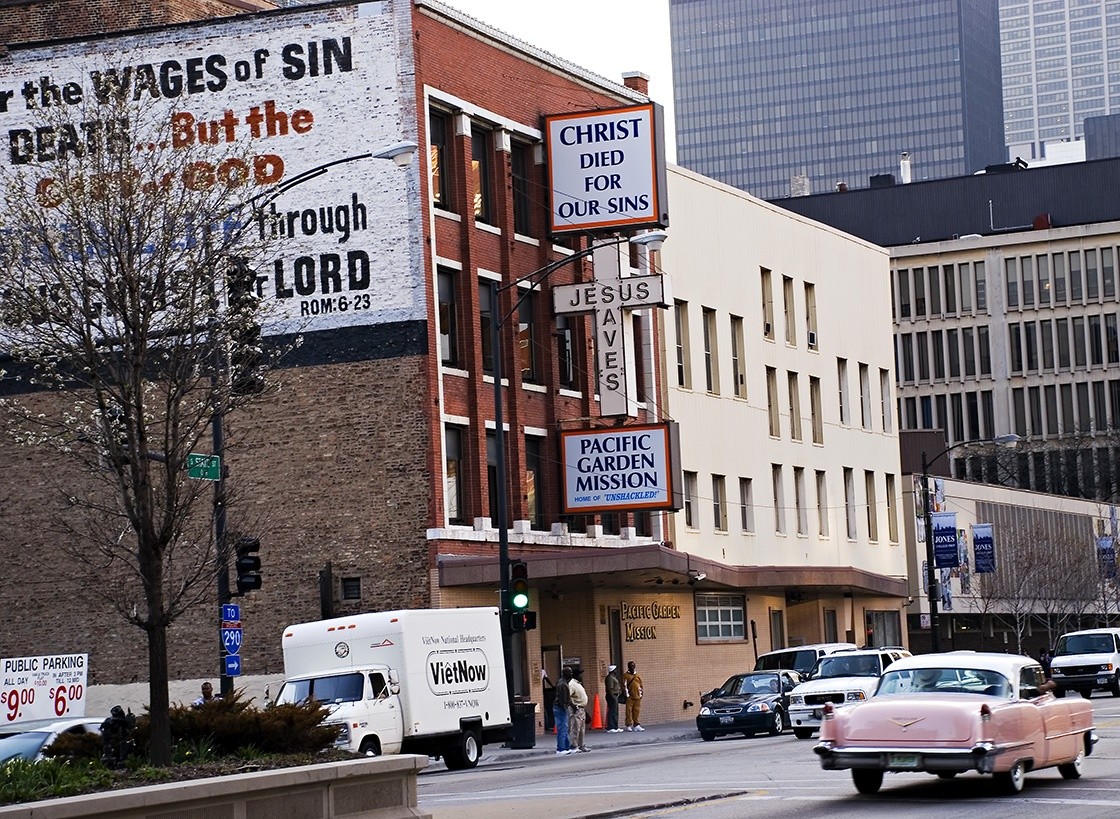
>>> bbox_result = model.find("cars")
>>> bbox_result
[696,667,807,741]
[0,715,111,771]
[814,648,1099,795]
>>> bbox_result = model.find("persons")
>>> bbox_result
[621,661,645,731]
[605,665,624,733]
[552,667,577,755]
[567,667,591,754]
[193,682,223,706]
[542,669,556,731]
[1022,648,1030,657]
[1038,648,1051,677]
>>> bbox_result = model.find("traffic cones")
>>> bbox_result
[590,694,603,729]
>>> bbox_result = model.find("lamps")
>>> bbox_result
[687,569,706,582]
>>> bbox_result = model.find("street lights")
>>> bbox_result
[920,433,1023,654]
[490,230,670,750]
[202,140,422,697]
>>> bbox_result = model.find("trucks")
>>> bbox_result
[269,605,511,771]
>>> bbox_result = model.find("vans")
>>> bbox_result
[752,643,858,683]
[1049,628,1120,698]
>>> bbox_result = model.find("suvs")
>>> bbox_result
[787,646,914,740]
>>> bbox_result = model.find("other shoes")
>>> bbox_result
[606,728,618,733]
[556,750,570,755]
[568,749,577,754]
[634,725,645,731]
[575,748,581,753]
[580,747,591,752]
[617,728,623,733]
[626,726,632,731]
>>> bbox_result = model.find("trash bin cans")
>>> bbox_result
[508,703,539,750]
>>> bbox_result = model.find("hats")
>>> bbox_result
[608,665,617,672]
[571,668,584,678]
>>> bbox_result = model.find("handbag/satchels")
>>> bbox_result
[618,691,627,704]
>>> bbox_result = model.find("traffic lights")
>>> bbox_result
[236,538,263,593]
[510,561,531,610]
[228,322,265,396]
[511,611,537,631]
